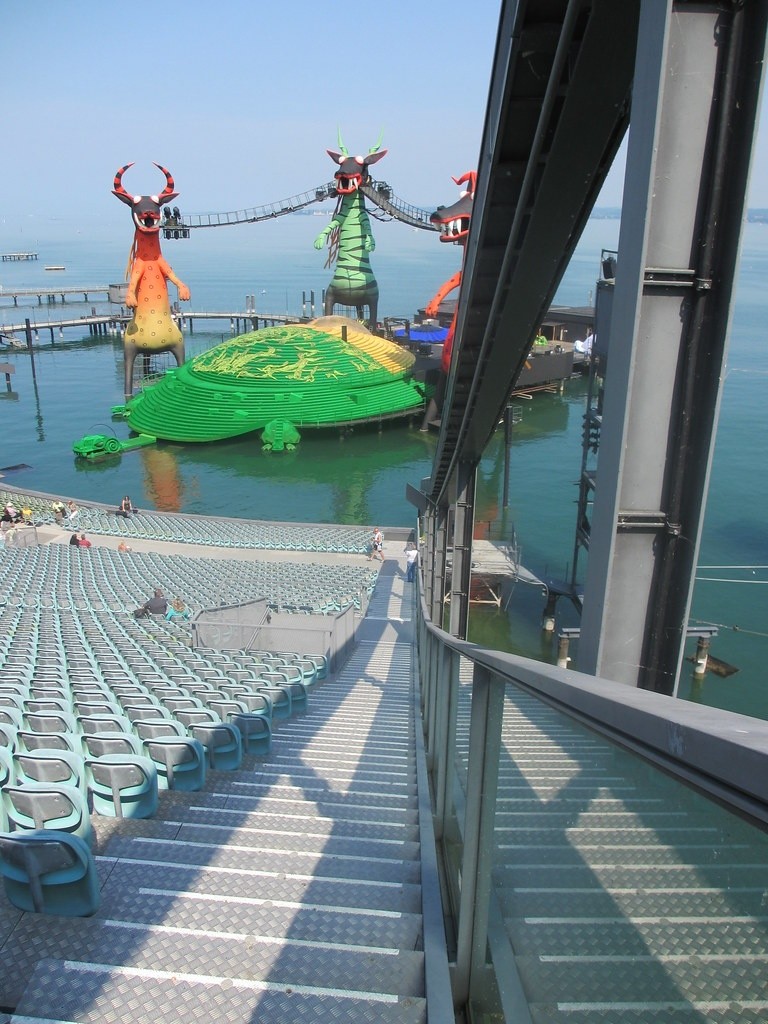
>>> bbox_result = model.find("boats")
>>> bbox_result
[45,267,65,270]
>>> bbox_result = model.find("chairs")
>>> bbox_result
[0,489,387,918]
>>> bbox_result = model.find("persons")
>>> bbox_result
[118,539,132,552]
[52,499,64,513]
[6,502,22,517]
[404,544,417,583]
[144,589,167,614]
[165,600,189,621]
[70,534,79,547]
[367,528,384,563]
[79,534,91,547]
[121,496,131,512]
[22,505,33,517]
[68,501,79,512]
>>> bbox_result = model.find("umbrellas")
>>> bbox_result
[396,326,450,356]
